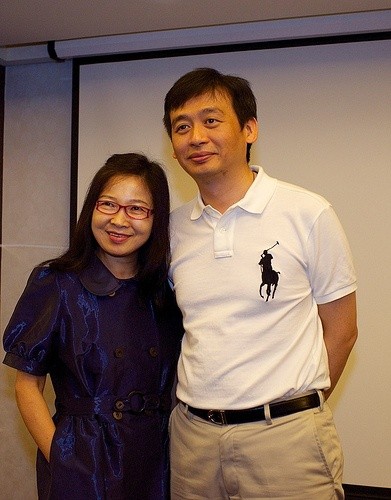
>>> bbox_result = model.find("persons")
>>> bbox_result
[0,67,359,500]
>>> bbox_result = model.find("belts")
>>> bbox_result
[182,393,320,426]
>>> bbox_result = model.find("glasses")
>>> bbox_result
[96,200,154,220]
